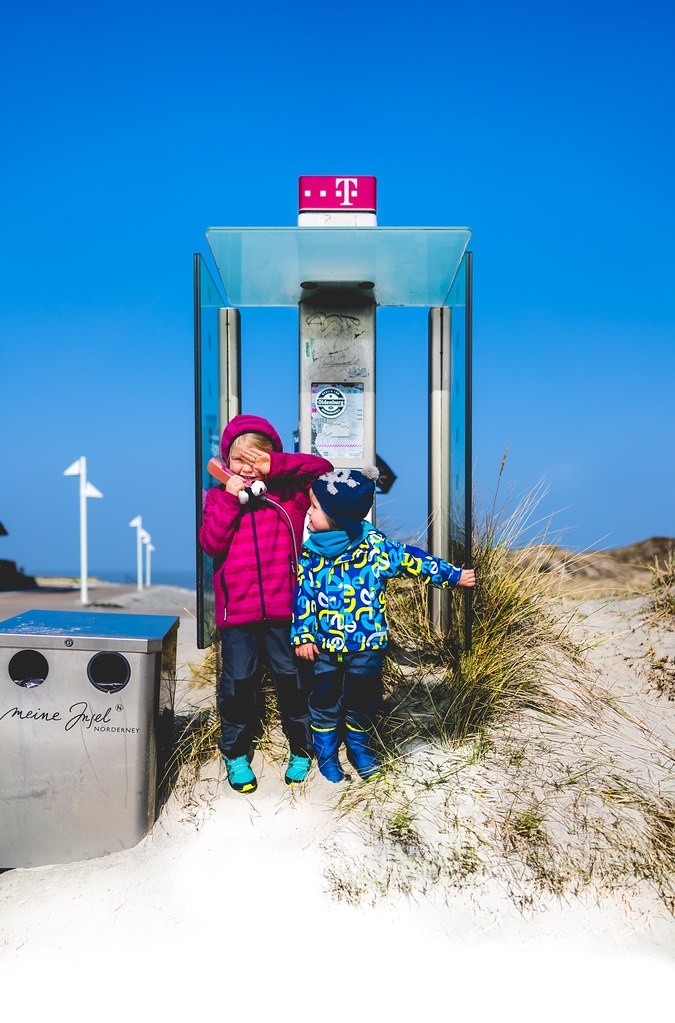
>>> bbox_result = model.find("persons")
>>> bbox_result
[291,465,475,785]
[199,413,334,794]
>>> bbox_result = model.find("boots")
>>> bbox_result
[310,724,352,783]
[345,724,380,778]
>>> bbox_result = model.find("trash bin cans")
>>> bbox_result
[0,610,183,873]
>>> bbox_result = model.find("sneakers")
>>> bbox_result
[222,754,257,792]
[285,753,311,787]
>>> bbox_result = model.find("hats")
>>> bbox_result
[311,467,379,535]
[221,414,284,461]
